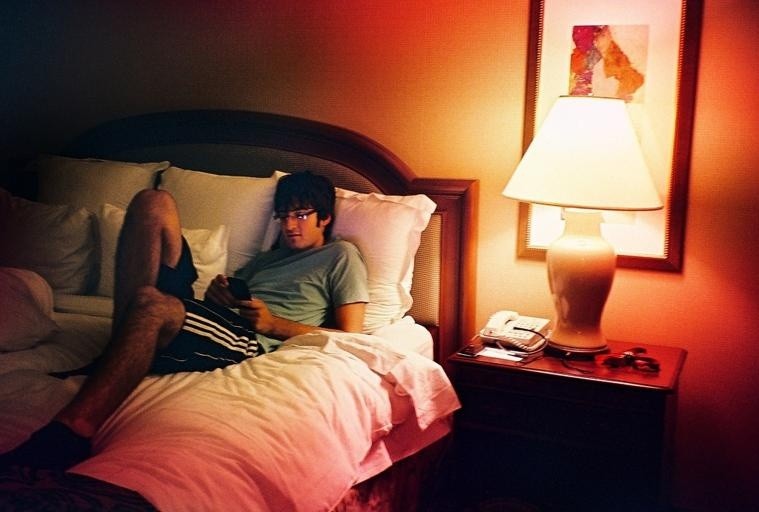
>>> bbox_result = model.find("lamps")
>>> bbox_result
[500,94,667,356]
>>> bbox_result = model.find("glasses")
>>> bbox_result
[271,210,316,224]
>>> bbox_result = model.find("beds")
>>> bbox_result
[1,108,479,511]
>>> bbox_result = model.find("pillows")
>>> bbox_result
[0,153,436,350]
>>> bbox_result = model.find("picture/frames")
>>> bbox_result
[518,0,703,274]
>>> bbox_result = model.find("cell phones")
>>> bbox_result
[227,277,251,300]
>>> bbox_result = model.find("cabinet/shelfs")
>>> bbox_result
[445,331,688,512]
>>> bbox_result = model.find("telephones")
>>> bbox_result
[480,310,552,348]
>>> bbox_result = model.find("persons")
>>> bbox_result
[0,170,366,471]
